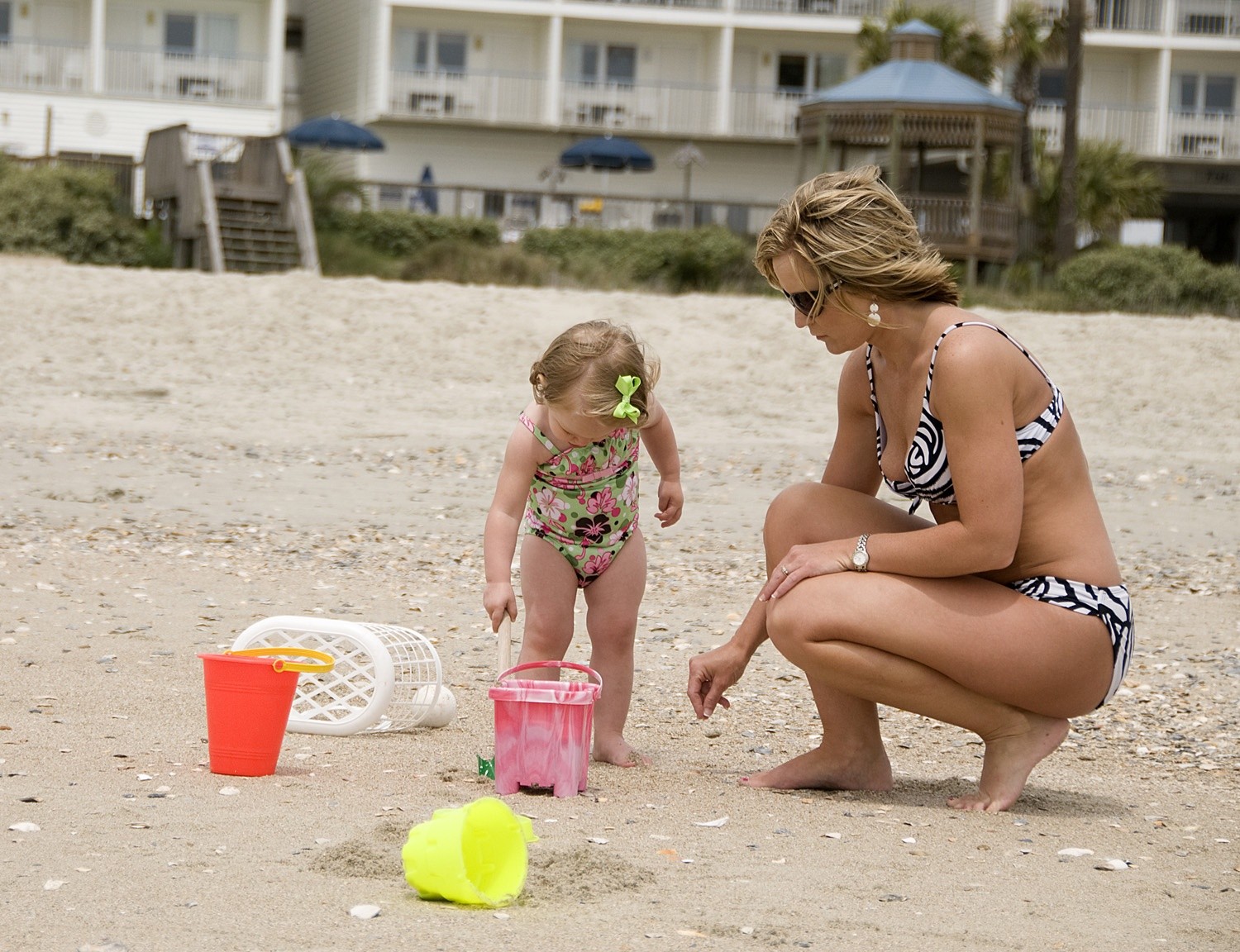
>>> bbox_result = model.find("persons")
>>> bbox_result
[481,318,683,767]
[686,162,1135,813]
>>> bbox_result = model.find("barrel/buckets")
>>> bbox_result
[199,647,335,776]
[403,797,537,908]
[488,659,603,798]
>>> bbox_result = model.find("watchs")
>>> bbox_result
[851,533,870,572]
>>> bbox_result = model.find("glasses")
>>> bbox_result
[781,278,844,317]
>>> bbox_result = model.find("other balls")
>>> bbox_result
[412,684,456,728]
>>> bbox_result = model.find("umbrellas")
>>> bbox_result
[560,135,656,175]
[283,117,385,151]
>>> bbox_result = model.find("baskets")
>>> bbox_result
[232,615,442,736]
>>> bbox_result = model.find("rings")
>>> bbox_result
[779,566,789,577]
